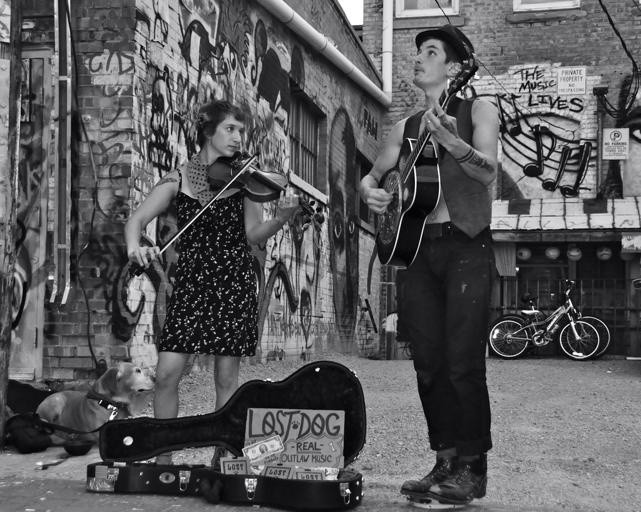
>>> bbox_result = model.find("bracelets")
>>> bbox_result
[455,146,475,163]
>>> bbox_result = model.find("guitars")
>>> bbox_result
[370,53,480,268]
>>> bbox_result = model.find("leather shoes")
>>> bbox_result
[400,453,488,505]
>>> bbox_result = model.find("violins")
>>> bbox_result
[207,154,325,228]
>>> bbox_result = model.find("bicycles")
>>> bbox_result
[488,278,613,360]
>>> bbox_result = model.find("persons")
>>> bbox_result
[359,24,498,503]
[125,102,299,471]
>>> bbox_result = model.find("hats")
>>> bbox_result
[414,26,477,72]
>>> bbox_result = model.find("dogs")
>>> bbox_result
[34,361,158,446]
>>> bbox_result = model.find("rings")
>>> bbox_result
[426,120,430,125]
